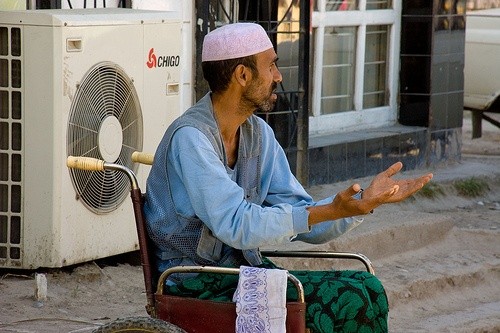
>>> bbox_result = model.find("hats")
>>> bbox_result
[202,22,273,60]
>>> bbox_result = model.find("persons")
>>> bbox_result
[144,23,433,333]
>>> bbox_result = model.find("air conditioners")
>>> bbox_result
[0,7,193,269]
